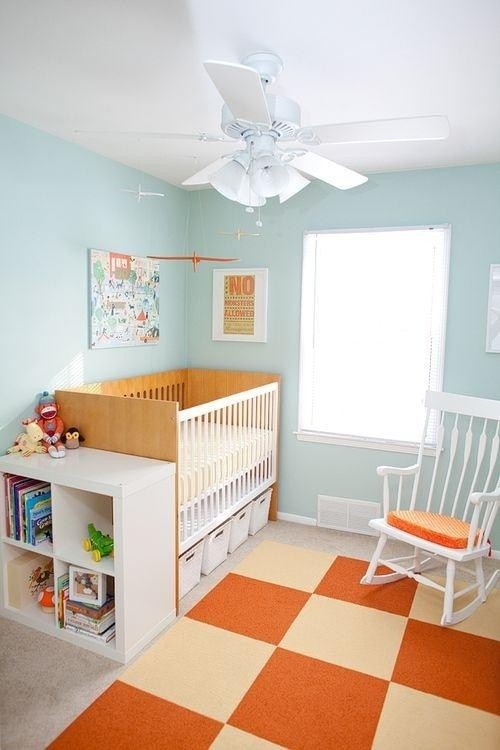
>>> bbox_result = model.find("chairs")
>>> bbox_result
[359,390,500,627]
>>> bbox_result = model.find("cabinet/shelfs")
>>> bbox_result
[1,445,177,666]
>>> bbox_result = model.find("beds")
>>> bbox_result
[55,366,283,618]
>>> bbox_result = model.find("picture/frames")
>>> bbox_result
[211,267,268,343]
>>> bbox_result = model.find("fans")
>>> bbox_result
[72,51,451,228]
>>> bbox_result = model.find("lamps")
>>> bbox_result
[209,136,310,207]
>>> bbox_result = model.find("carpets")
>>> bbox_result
[44,539,499,749]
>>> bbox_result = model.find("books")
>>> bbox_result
[57,564,115,643]
[4,473,53,546]
[7,551,54,609]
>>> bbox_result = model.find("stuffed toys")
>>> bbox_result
[6,391,85,458]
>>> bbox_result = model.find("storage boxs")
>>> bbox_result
[177,488,275,602]
